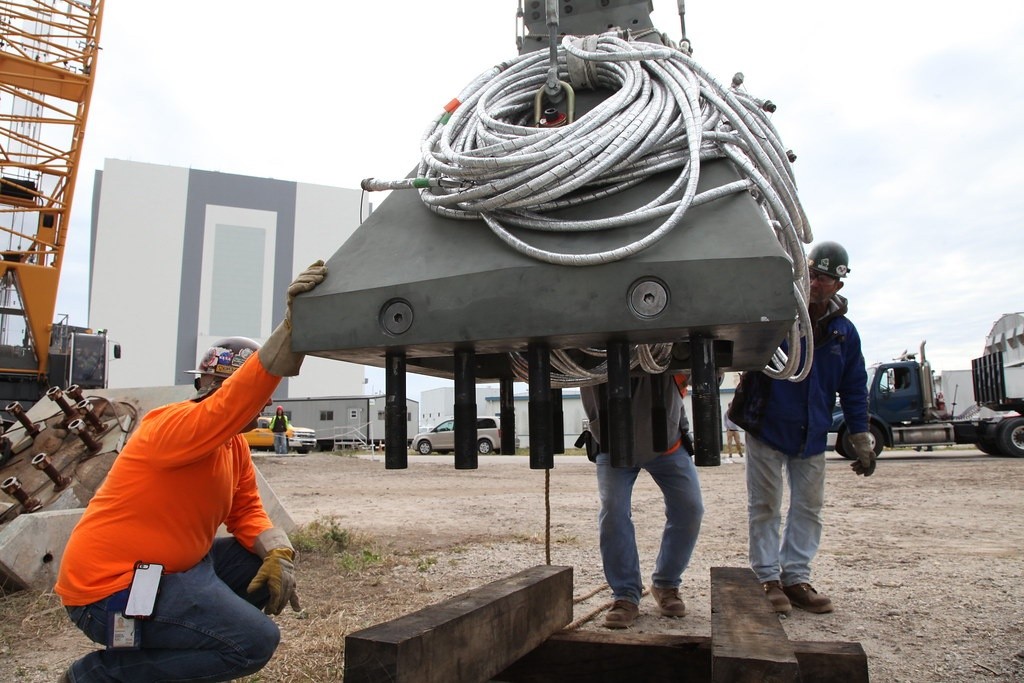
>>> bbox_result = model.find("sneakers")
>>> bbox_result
[651,584,685,616]
[604,600,639,627]
[760,580,792,611]
[783,583,833,613]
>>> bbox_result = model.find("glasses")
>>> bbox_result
[810,272,839,285]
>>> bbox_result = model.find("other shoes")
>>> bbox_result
[913,448,933,451]
[58,670,71,683]
[729,453,744,458]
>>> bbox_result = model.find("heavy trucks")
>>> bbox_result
[826,313,1024,458]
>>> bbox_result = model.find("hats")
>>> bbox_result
[277,406,282,410]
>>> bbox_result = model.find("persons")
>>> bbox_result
[579,371,723,628]
[269,405,290,453]
[724,402,743,458]
[727,241,877,614]
[53,258,328,683]
[912,446,933,452]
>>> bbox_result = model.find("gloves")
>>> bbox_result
[848,432,876,477]
[247,528,300,615]
[258,261,327,377]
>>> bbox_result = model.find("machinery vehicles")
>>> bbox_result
[0,0,121,417]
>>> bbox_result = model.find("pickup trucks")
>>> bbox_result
[245,417,317,455]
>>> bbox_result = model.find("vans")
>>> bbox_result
[412,416,519,455]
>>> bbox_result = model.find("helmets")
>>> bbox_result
[183,337,261,377]
[807,241,850,277]
